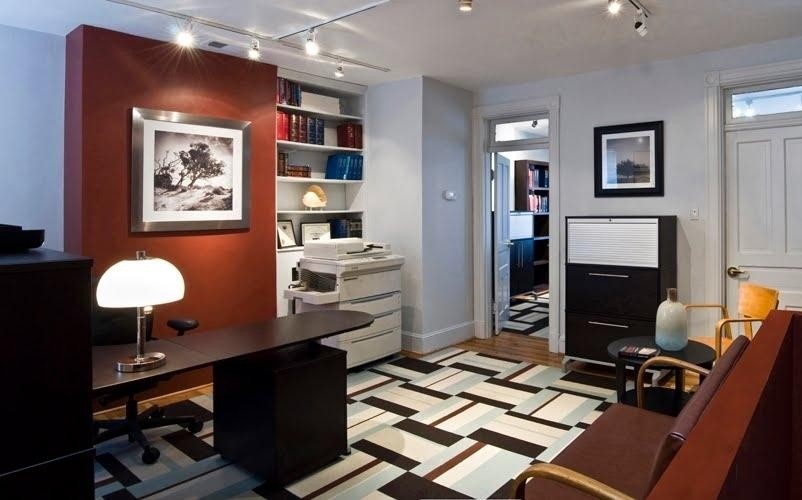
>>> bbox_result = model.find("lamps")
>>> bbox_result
[107,0,390,75]
[607,0,651,37]
[532,120,537,128]
[273,0,472,44]
[97,251,186,373]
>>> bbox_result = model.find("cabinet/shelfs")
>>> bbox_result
[515,160,548,285]
[491,212,538,301]
[0,248,95,500]
[562,215,677,389]
[276,64,367,317]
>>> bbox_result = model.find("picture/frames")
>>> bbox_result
[129,107,251,232]
[594,120,664,197]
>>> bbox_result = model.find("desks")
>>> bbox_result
[607,336,716,418]
[92,310,372,486]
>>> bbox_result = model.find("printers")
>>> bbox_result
[283,238,404,370]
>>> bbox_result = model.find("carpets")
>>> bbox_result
[491,288,548,340]
[94,345,650,500]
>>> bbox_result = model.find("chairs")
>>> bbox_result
[683,285,780,375]
[509,309,802,500]
[93,305,203,464]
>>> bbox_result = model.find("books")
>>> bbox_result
[528,165,549,213]
[618,342,660,360]
[328,218,362,238]
[276,77,364,181]
